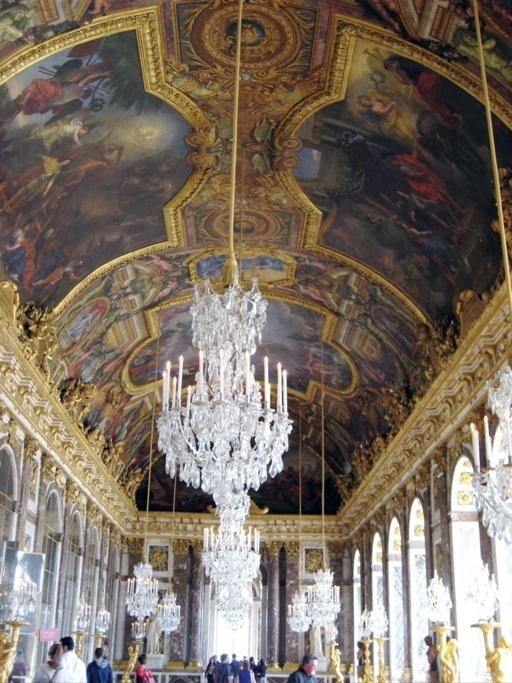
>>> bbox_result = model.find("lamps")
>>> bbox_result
[465,364,511,543]
[3,541,510,683]
[150,254,298,532]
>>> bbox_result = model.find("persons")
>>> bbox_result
[286,652,319,683]
[422,635,437,682]
[204,652,267,682]
[60,636,88,683]
[136,653,157,682]
[356,641,366,677]
[33,643,69,683]
[86,646,114,682]
